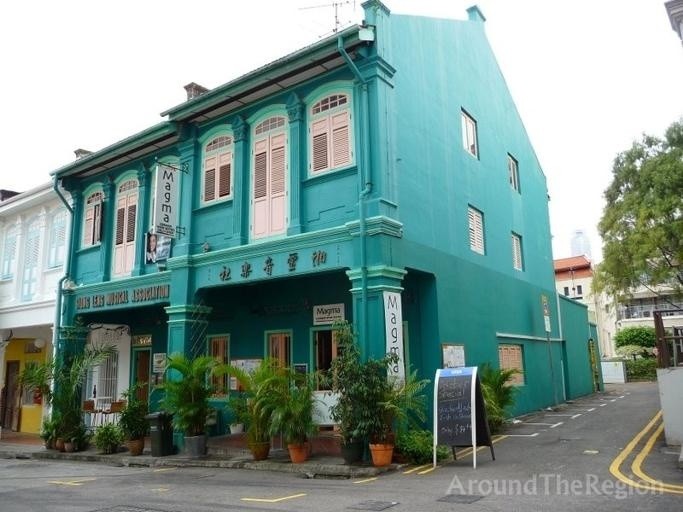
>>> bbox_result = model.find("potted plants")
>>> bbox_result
[14,319,432,469]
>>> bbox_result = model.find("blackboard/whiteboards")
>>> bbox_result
[434,365,478,447]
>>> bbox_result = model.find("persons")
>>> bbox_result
[146,234,157,264]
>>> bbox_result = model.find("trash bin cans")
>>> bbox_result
[144,410,173,457]
